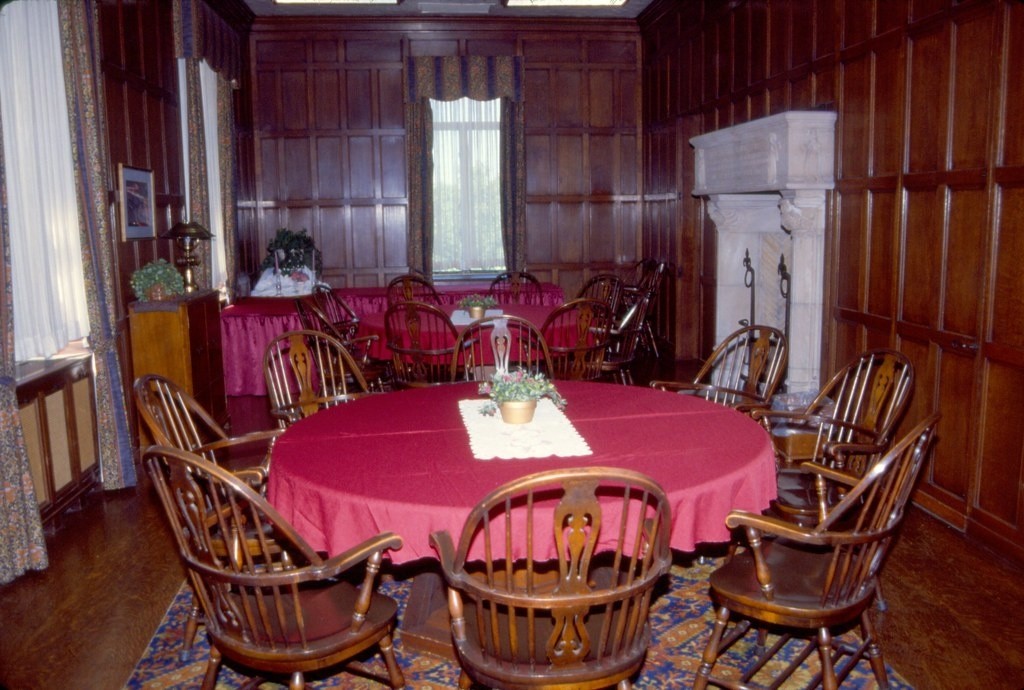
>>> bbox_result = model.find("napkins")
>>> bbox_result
[451,310,503,326]
[458,397,593,460]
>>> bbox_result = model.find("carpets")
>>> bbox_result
[126,544,913,690]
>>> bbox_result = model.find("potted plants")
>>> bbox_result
[130,259,185,304]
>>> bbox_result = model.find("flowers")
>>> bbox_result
[456,293,498,315]
[476,364,568,416]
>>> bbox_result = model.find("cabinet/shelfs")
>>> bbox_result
[128,289,228,478]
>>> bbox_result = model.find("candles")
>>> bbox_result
[312,249,315,271]
[273,251,279,275]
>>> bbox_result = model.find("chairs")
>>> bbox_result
[649,325,789,413]
[132,373,295,664]
[490,271,544,305]
[263,329,371,428]
[623,257,668,357]
[694,411,940,690]
[294,297,386,392]
[750,350,918,529]
[383,301,480,391]
[142,445,408,690]
[311,283,360,355]
[571,285,656,386]
[427,466,673,690]
[518,297,613,383]
[386,274,443,308]
[450,315,555,383]
[576,273,625,316]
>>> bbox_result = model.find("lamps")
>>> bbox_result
[161,221,217,294]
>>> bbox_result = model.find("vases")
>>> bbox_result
[468,306,486,319]
[497,400,538,424]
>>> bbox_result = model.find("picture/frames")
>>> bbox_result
[117,163,158,243]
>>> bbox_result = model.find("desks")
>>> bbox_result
[220,282,779,665]
